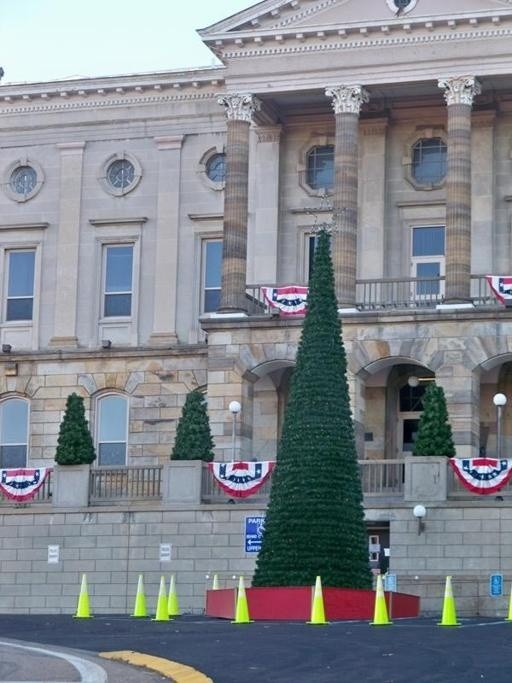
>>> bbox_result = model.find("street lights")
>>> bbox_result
[492,392,507,458]
[228,399,243,462]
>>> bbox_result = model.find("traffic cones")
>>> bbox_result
[230,574,256,625]
[70,572,93,619]
[168,574,184,617]
[435,574,465,625]
[128,572,152,618]
[150,574,174,622]
[502,591,512,621]
[368,574,394,626]
[305,573,330,625]
[212,573,221,589]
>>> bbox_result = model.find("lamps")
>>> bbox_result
[412,504,426,537]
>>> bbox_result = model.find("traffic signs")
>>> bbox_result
[244,515,267,554]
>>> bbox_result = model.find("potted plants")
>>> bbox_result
[161,387,218,505]
[397,380,458,501]
[52,390,99,509]
